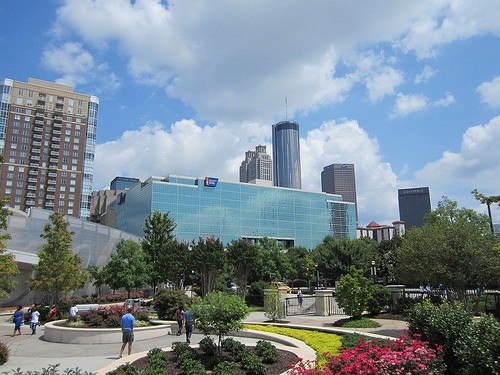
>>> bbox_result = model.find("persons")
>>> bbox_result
[176,305,184,336]
[30,308,40,335]
[297,287,303,305]
[184,307,193,343]
[418,282,481,301]
[118,308,136,359]
[48,303,57,321]
[70,304,78,317]
[12,305,24,337]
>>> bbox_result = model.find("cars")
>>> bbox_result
[230,283,241,292]
[268,282,290,294]
